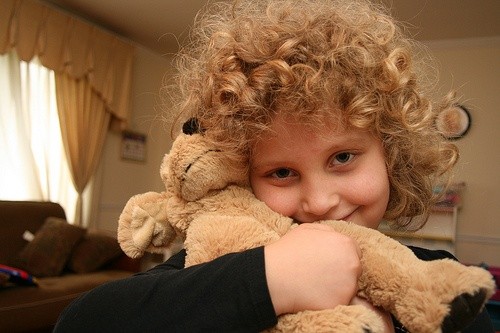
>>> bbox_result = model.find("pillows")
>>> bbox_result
[23,215,123,276]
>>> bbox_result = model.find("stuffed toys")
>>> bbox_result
[118,134,495,333]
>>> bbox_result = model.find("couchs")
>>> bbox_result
[0,201,136,333]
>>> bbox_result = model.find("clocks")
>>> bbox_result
[433,105,471,141]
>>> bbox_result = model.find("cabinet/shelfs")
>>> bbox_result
[377,206,457,258]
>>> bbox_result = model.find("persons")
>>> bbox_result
[54,0,497,333]
